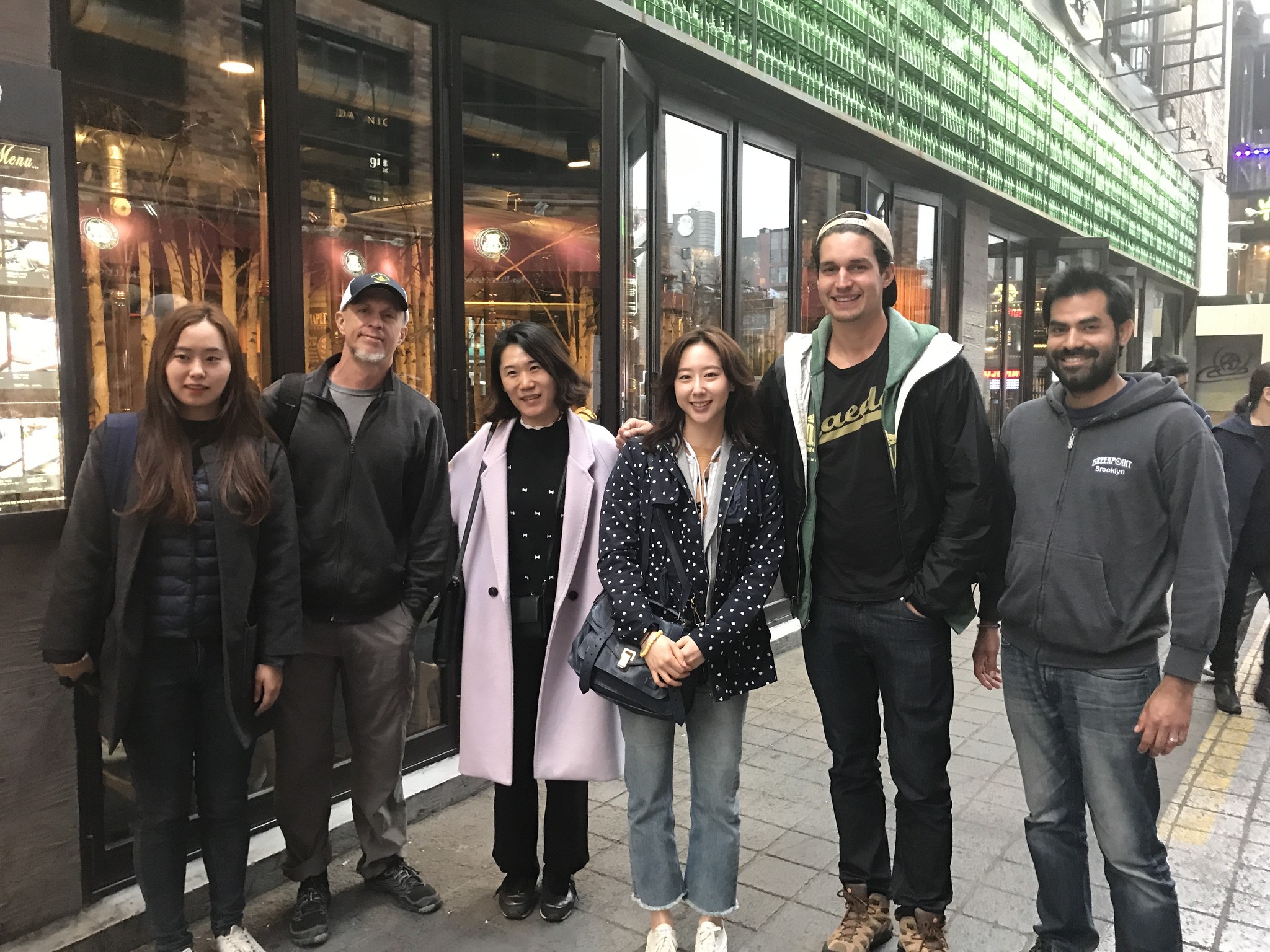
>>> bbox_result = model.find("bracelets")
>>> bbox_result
[639,629,664,658]
[977,622,1000,628]
[53,655,87,668]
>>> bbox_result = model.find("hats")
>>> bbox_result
[815,211,898,308]
[339,271,410,327]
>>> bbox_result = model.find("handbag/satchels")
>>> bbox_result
[568,589,694,724]
[427,573,464,667]
[55,409,138,694]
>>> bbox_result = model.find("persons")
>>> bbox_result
[253,274,444,945]
[612,210,997,952]
[439,322,620,923]
[969,268,1270,952]
[601,328,786,952]
[37,304,301,952]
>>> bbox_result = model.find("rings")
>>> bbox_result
[1169,736,1179,742]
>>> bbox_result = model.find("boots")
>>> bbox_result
[1213,669,1242,713]
[1255,664,1270,713]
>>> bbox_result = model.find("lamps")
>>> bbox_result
[1070,25,1230,186]
[216,24,255,82]
[564,132,594,172]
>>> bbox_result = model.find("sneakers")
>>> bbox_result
[208,927,265,952]
[897,906,948,952]
[288,875,330,946]
[364,862,443,915]
[645,922,679,952]
[539,871,580,922]
[493,865,539,919]
[694,921,728,952]
[823,882,893,952]
